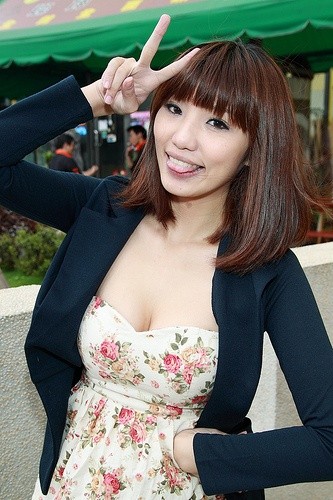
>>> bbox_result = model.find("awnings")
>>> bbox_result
[0,0,333,101]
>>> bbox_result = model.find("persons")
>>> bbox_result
[1,11,331,500]
[125,125,147,173]
[47,133,98,178]
